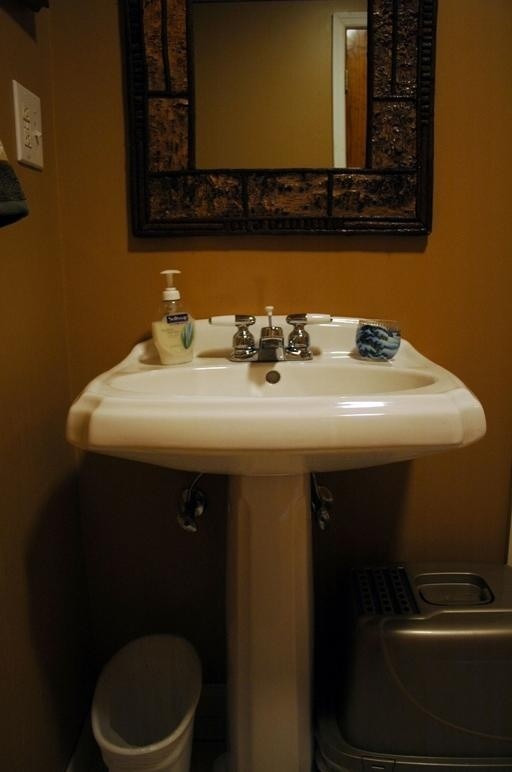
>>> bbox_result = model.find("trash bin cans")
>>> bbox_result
[92,634,202,772]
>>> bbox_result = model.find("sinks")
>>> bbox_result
[68,313,487,478]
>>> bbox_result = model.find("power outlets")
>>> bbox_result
[10,78,49,174]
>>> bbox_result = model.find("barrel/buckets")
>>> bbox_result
[93,634,202,772]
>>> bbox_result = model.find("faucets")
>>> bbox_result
[258,304,286,361]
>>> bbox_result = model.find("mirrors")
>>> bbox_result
[117,1,436,240]
